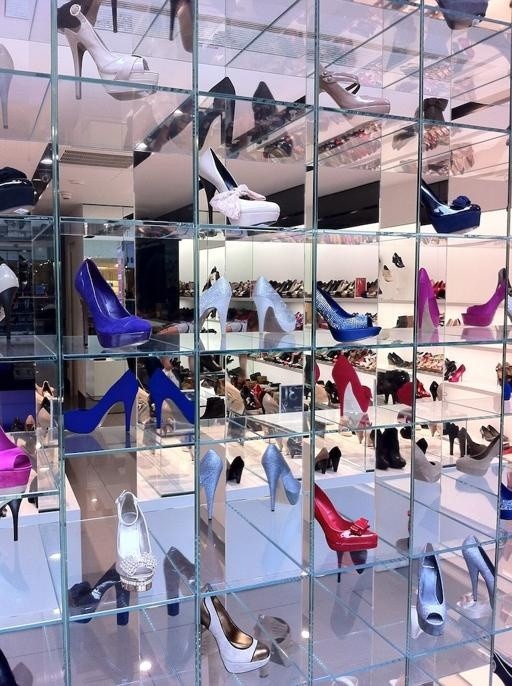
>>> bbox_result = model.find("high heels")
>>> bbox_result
[200,450,223,519]
[169,0,193,53]
[0,262,19,341]
[0,498,22,542]
[164,547,198,616]
[179,267,512,483]
[312,481,377,551]
[149,370,195,428]
[198,77,235,151]
[461,534,496,608]
[116,489,156,591]
[421,178,482,234]
[0,425,33,473]
[75,258,151,349]
[0,43,13,129]
[262,444,301,512]
[64,4,159,101]
[66,562,130,625]
[64,370,138,434]
[416,543,448,636]
[193,584,272,673]
[318,62,390,113]
[197,147,281,226]
[57,0,118,33]
[250,81,293,158]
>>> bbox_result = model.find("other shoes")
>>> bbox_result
[0,167,36,216]
[435,0,488,30]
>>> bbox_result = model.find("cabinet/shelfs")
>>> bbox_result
[1,0,511,686]
